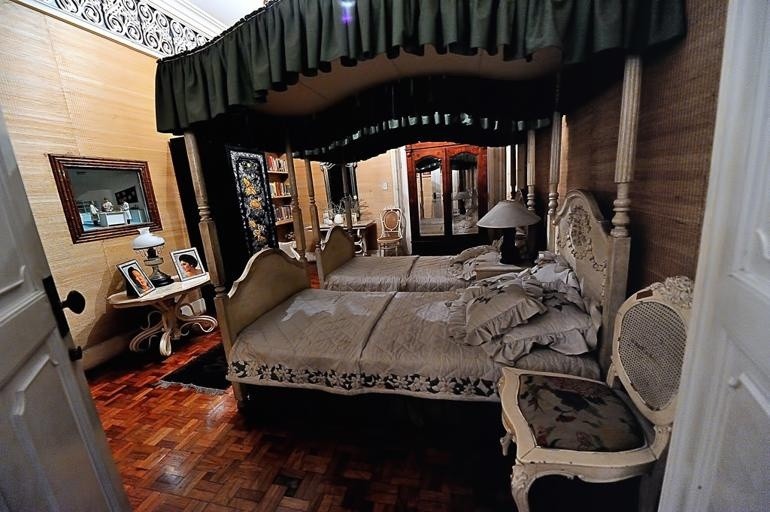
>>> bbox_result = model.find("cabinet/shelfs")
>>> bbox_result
[265,152,297,248]
[75,200,85,214]
[474,263,522,281]
[406,141,489,255]
[83,200,97,214]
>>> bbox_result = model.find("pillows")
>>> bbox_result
[464,272,518,287]
[447,234,504,263]
[481,279,604,368]
[453,252,505,277]
[530,250,578,296]
[444,269,546,347]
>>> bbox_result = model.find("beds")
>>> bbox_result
[302,116,562,292]
[155,0,688,412]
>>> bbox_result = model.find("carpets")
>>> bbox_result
[159,342,230,393]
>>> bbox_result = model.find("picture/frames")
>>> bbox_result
[117,258,155,298]
[168,246,206,282]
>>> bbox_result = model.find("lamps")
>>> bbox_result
[476,198,543,265]
[131,223,174,287]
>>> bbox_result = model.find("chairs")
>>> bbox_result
[376,207,402,256]
[496,273,695,512]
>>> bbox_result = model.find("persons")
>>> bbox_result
[88,199,100,226]
[178,253,202,277]
[102,197,114,212]
[120,199,132,224]
[127,266,151,293]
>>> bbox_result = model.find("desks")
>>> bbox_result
[105,270,217,354]
[304,218,376,258]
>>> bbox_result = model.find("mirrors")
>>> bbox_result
[414,154,445,236]
[449,150,478,234]
[319,160,360,222]
